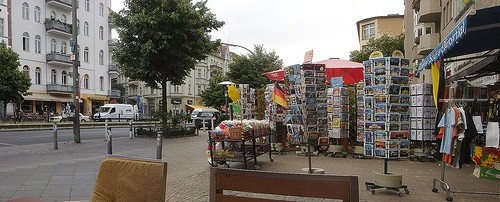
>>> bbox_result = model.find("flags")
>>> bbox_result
[265,70,287,108]
[429,60,440,107]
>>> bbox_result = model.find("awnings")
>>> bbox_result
[20,92,83,102]
[187,104,207,109]
[417,6,500,75]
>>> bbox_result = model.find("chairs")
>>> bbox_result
[90,157,168,202]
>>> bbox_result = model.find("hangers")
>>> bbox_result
[445,98,471,115]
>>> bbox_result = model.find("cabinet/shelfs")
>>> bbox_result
[356,83,364,144]
[209,123,274,169]
[327,88,350,156]
[412,83,438,160]
[282,64,323,173]
[363,58,411,196]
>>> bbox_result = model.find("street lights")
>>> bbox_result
[219,41,255,59]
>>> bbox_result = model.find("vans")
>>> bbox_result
[92,103,135,123]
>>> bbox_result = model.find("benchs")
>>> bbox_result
[209,167,359,202]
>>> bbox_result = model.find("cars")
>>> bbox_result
[49,111,90,123]
[189,108,220,129]
